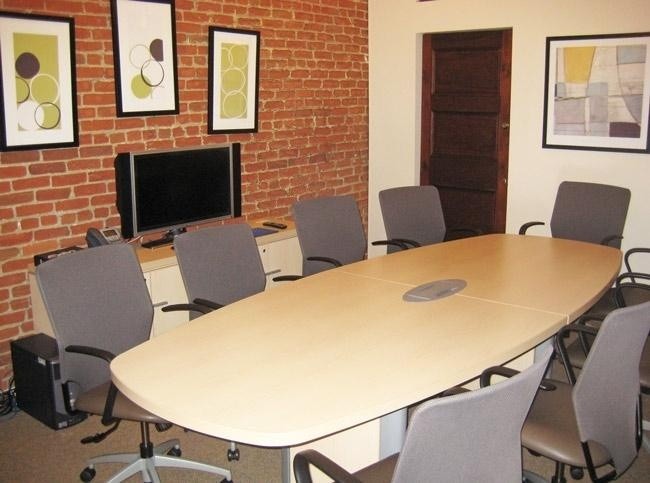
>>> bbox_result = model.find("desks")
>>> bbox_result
[110,234,623,483]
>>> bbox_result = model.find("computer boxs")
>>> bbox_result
[10,333,89,430]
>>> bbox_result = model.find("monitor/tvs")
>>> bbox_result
[115,141,241,251]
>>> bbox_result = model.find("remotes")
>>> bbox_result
[263,221,287,229]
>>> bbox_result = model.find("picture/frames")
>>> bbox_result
[110,0,179,117]
[0,11,79,152]
[542,33,650,153]
[208,26,260,134]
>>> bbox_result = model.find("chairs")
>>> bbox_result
[481,300,649,483]
[519,181,631,249]
[174,223,305,321]
[585,248,650,328]
[291,196,408,278]
[36,245,233,483]
[293,345,554,483]
[379,186,480,254]
[559,283,650,430]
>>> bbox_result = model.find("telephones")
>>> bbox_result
[85,226,121,248]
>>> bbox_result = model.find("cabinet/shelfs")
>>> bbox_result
[28,219,303,339]
